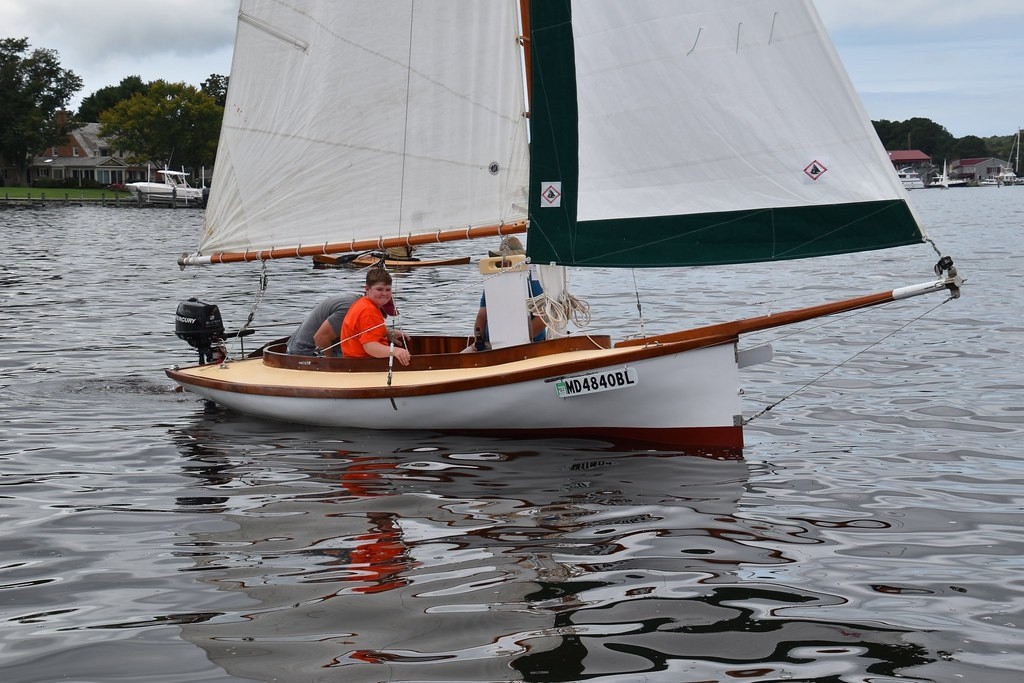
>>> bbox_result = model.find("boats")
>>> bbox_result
[304,251,473,271]
[123,164,210,208]
[897,159,970,189]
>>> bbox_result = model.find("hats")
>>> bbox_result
[488,236,526,257]
[381,297,400,316]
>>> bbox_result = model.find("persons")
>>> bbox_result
[376,244,417,260]
[340,269,411,366]
[459,236,546,353]
[285,291,401,357]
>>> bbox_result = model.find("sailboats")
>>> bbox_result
[164,0,968,451]
[981,126,1024,185]
